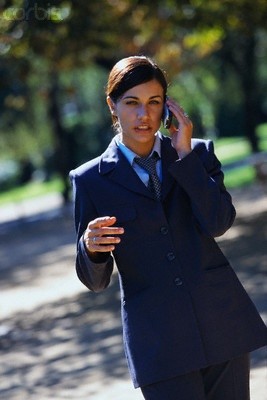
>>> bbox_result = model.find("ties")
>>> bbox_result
[134,154,161,198]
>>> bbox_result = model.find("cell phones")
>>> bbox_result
[160,96,172,127]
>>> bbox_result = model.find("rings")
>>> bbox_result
[92,237,96,244]
[183,113,189,119]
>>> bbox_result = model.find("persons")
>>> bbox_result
[68,55,267,399]
